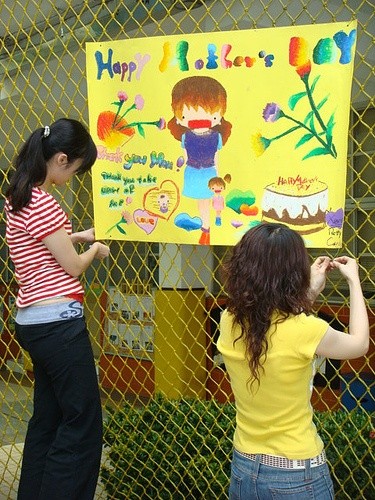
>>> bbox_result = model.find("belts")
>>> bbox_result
[232,447,328,470]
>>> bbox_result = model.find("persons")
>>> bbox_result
[216,223,371,500]
[4,118,110,500]
